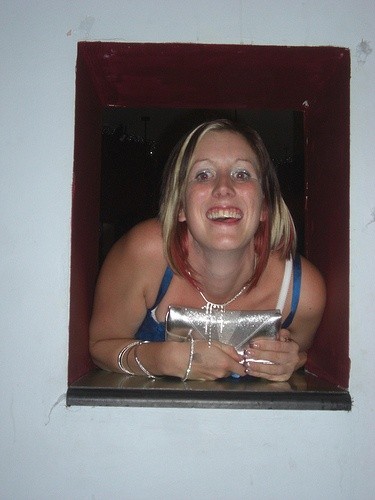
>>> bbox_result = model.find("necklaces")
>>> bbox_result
[186,267,258,348]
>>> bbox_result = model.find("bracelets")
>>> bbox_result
[117,340,166,380]
[180,334,195,383]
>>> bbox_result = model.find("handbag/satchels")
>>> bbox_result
[164,305,282,364]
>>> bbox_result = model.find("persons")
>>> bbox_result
[89,119,328,383]
